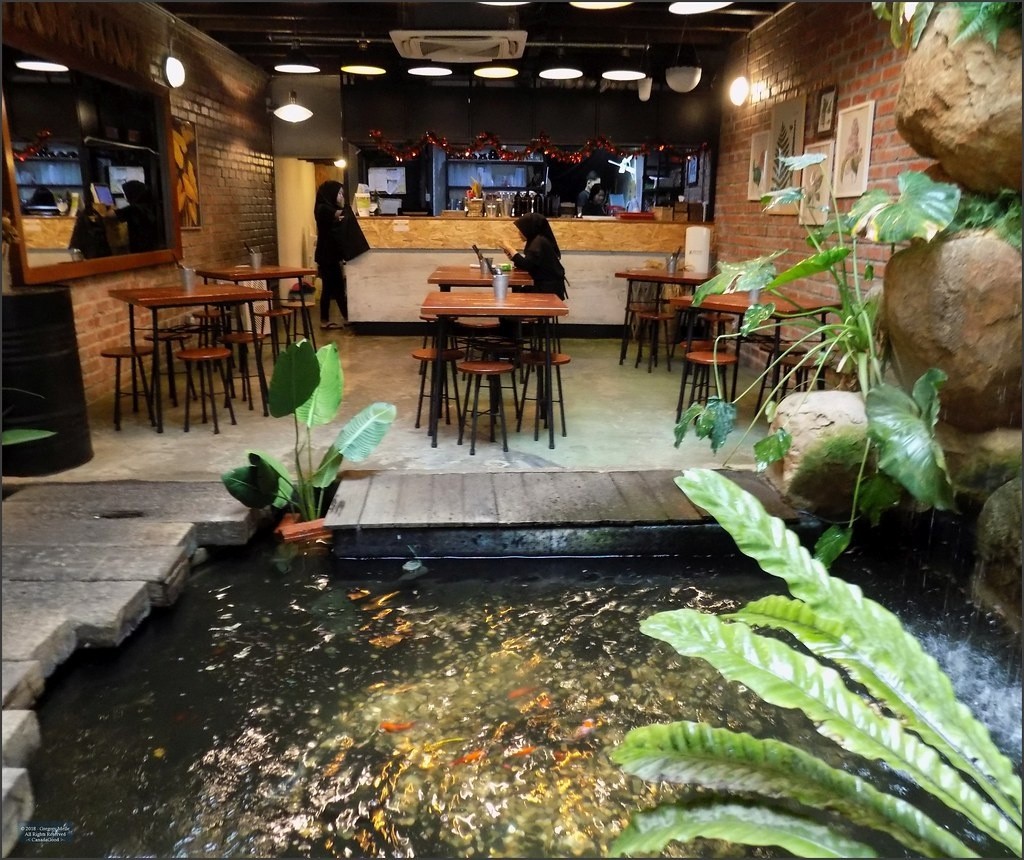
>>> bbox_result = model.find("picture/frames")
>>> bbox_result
[813,84,839,138]
[798,139,835,226]
[767,95,806,216]
[686,156,699,185]
[831,99,875,197]
[747,130,770,201]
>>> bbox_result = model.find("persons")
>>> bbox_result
[575,171,610,216]
[24,186,61,215]
[315,180,349,329]
[107,181,160,254]
[498,212,570,368]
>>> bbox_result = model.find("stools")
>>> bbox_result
[104,298,825,456]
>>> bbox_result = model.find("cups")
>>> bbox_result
[355,193,370,216]
[179,269,196,292]
[478,257,494,276]
[250,254,262,268]
[667,257,677,274]
[492,274,509,300]
[748,288,760,306]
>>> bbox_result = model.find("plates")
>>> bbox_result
[470,264,496,268]
[235,265,251,267]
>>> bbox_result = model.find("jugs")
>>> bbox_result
[625,198,641,213]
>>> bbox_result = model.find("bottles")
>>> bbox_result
[484,190,561,219]
[447,196,468,211]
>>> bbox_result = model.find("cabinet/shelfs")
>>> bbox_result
[13,154,82,190]
[444,143,547,211]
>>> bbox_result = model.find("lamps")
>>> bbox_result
[729,76,749,106]
[275,2,735,122]
[163,56,187,89]
[16,32,69,72]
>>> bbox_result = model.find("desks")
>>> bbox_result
[194,265,316,373]
[666,291,843,411]
[428,265,534,348]
[107,284,267,417]
[423,293,568,436]
[615,269,720,366]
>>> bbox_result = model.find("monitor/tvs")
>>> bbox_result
[88,183,116,206]
